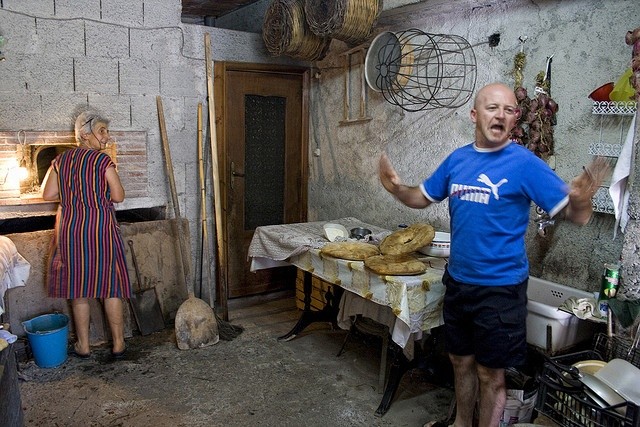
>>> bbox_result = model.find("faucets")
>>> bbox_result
[533,212,557,238]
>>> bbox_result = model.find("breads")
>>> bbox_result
[364,255,427,275]
[321,242,379,261]
[380,224,435,255]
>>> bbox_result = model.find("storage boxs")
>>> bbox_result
[536,349,640,427]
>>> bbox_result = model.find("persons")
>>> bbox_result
[378,82,610,426]
[42,110,130,356]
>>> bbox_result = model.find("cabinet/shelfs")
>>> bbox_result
[584,101,636,215]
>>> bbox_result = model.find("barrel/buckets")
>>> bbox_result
[22,312,71,369]
[479,378,538,427]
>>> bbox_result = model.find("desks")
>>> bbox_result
[249,217,447,419]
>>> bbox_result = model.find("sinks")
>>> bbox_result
[509,270,596,353]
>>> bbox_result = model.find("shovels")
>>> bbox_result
[127,240,166,335]
[156,95,219,350]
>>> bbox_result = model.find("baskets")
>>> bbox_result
[304,0,384,48]
[261,0,332,62]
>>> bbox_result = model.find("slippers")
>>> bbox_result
[112,339,130,358]
[422,420,449,427]
[68,340,91,359]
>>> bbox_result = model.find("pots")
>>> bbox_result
[532,346,627,414]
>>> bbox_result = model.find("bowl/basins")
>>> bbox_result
[418,231,451,258]
[594,358,639,406]
[587,82,614,104]
